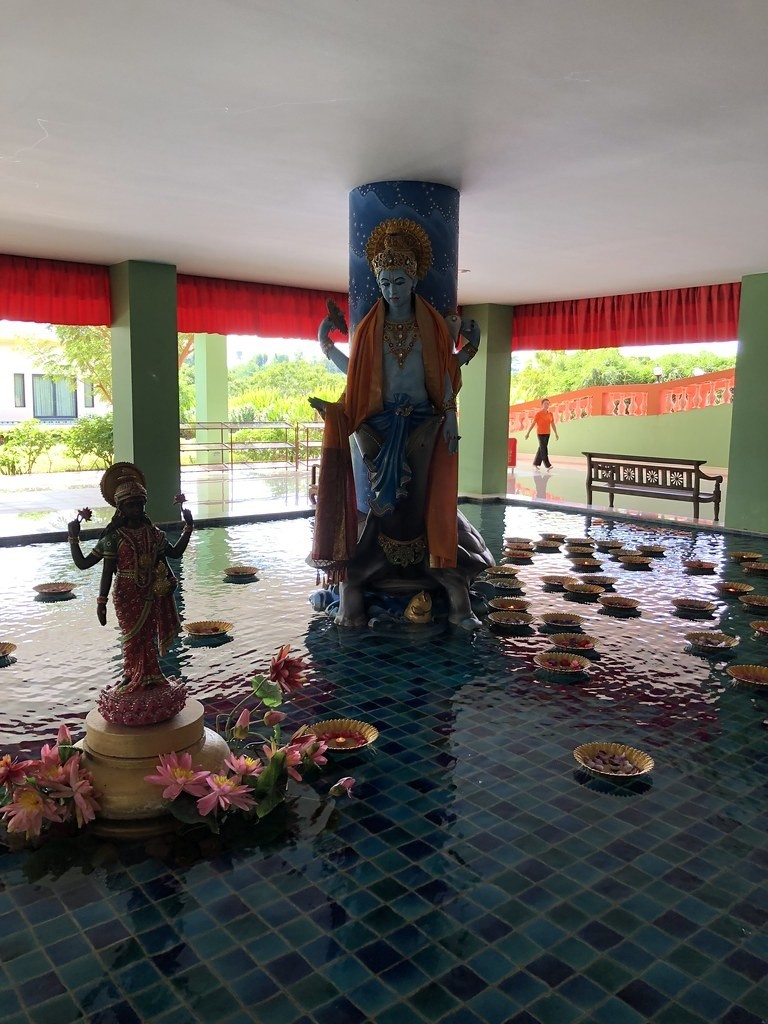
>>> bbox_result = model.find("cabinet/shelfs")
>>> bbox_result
[298,422,326,471]
[180,422,229,472]
[223,421,299,475]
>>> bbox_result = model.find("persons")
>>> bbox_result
[525,399,559,470]
[67,461,194,691]
[308,218,480,528]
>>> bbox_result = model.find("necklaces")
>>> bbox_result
[383,320,420,367]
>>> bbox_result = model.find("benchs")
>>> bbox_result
[308,463,321,505]
[581,452,723,521]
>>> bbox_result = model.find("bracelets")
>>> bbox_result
[170,577,178,580]
[96,597,108,604]
[321,339,333,360]
[463,343,478,357]
[442,402,456,411]
[184,525,192,533]
[68,537,79,544]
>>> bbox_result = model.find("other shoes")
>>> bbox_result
[536,466,541,471]
[547,465,553,471]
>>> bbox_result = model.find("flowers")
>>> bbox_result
[142,642,357,827]
[173,494,186,511]
[0,723,104,840]
[78,507,92,523]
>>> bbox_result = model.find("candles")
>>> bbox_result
[336,737,346,746]
[508,605,514,609]
